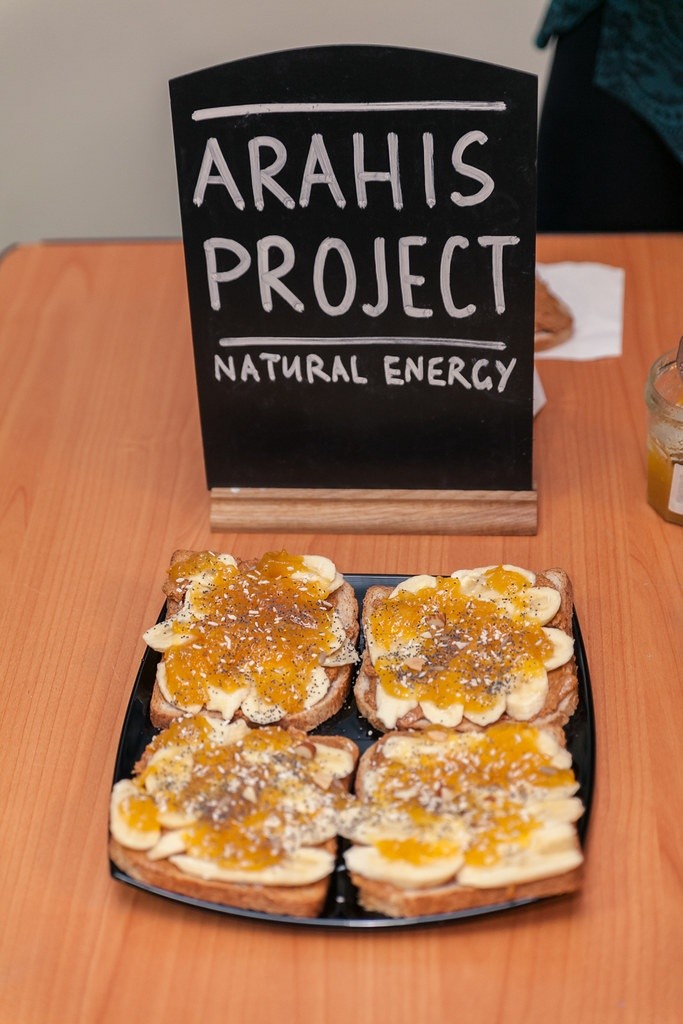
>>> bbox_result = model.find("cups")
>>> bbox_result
[643,348,683,529]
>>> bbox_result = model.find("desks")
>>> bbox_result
[1,236,683,1023]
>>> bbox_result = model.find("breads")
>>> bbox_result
[350,726,583,919]
[355,568,578,731]
[149,557,358,728]
[535,271,574,349]
[109,728,359,918]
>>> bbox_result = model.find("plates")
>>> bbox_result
[107,572,597,928]
